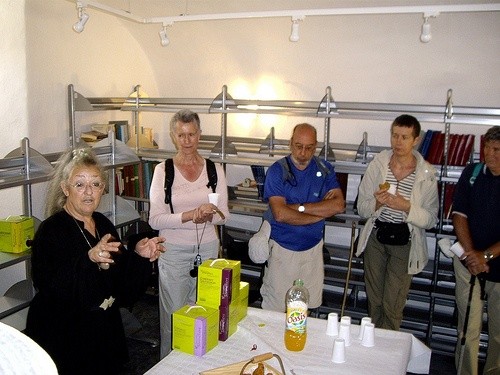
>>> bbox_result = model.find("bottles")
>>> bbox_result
[285,279,310,351]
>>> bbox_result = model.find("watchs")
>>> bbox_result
[298,203,305,214]
[484,249,493,261]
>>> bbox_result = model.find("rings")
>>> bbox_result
[99,252,104,256]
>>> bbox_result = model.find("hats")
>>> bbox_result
[249,220,271,263]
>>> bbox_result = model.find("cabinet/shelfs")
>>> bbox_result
[0,85,500,359]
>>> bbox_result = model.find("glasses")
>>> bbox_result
[67,182,105,193]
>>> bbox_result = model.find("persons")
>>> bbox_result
[357,115,440,330]
[26,145,167,375]
[148,110,230,362]
[260,123,344,313]
[453,127,500,375]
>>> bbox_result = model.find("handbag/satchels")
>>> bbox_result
[375,219,412,246]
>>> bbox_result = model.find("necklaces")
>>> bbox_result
[67,206,102,271]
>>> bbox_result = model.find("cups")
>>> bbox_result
[449,242,467,260]
[360,323,375,348]
[209,193,219,206]
[339,324,350,347]
[331,339,345,363]
[326,312,339,337]
[340,316,351,325]
[359,317,371,339]
[387,184,396,195]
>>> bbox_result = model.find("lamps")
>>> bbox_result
[289,15,304,41]
[73,2,89,32]
[159,23,170,45]
[421,12,439,42]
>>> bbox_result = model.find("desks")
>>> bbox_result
[141,307,431,375]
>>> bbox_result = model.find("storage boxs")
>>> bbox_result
[196,258,241,305]
[172,304,219,356]
[0,217,34,253]
[219,298,238,341]
[238,281,249,321]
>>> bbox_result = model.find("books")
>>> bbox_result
[415,129,490,220]
[234,181,260,202]
[81,121,160,199]
